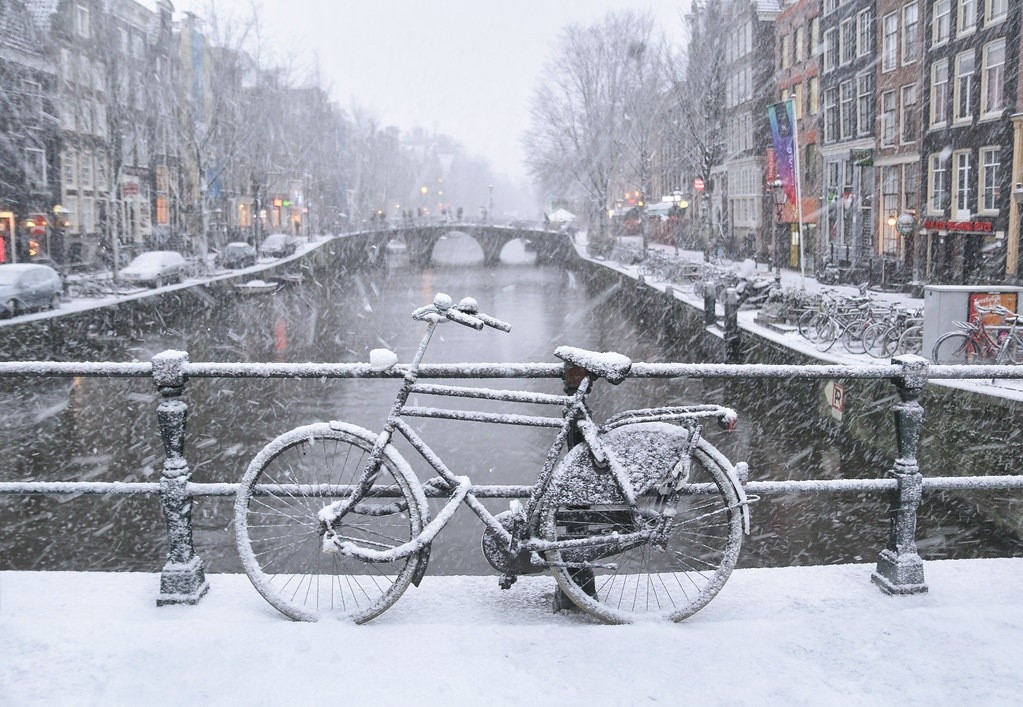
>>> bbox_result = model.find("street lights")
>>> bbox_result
[487,185,495,225]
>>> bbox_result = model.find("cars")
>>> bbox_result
[213,243,256,268]
[0,263,64,319]
[258,234,296,259]
[119,251,186,288]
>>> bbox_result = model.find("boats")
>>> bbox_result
[232,272,304,295]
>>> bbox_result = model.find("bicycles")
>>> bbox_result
[234,292,761,623]
[586,234,924,358]
[66,265,119,301]
[932,302,1023,383]
